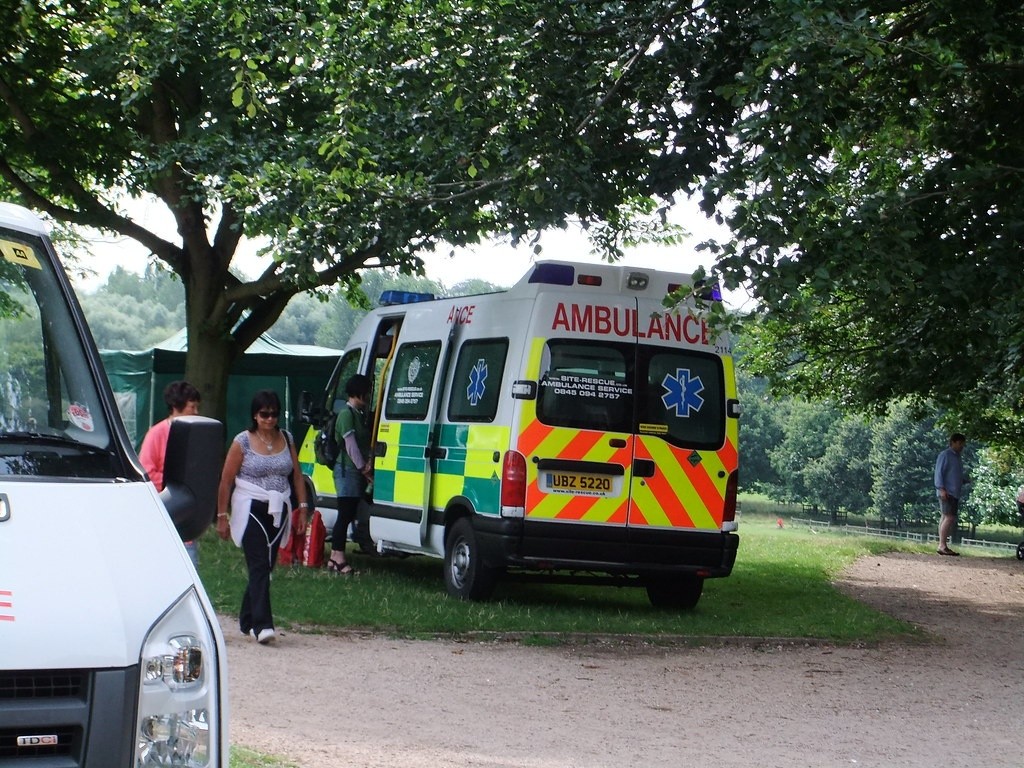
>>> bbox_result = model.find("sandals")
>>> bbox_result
[327,559,356,575]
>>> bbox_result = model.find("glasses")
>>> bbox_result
[259,410,279,418]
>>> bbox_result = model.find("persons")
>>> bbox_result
[326,375,371,577]
[935,434,965,555]
[137,379,201,570]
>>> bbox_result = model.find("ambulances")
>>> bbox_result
[297,259,740,611]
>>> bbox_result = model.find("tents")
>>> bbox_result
[99,309,347,458]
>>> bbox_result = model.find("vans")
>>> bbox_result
[1,201,229,768]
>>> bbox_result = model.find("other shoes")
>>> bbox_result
[258,628,275,643]
[937,547,959,556]
[249,628,258,642]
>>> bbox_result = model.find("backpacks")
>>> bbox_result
[277,509,326,568]
[314,405,355,477]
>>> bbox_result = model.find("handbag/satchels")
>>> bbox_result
[282,429,298,511]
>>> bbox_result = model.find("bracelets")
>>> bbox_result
[217,512,227,517]
[217,389,308,643]
[298,503,308,510]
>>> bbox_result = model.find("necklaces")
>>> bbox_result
[255,428,275,451]
